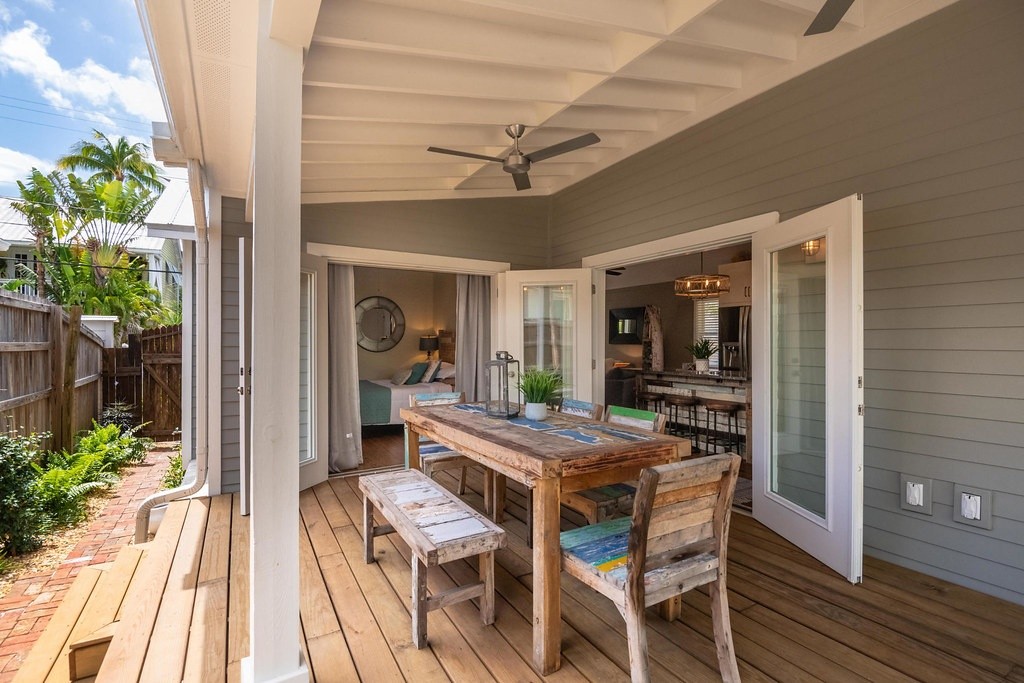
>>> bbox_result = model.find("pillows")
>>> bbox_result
[392,359,456,386]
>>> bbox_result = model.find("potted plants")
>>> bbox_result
[679,339,720,372]
[513,365,563,420]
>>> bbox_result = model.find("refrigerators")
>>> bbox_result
[718,306,752,377]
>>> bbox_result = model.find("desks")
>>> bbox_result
[400,400,691,677]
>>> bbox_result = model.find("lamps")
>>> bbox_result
[419,334,438,360]
[801,237,826,264]
[674,252,730,299]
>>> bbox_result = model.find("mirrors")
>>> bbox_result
[355,297,405,352]
[608,307,645,344]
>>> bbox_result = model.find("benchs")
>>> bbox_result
[359,467,508,651]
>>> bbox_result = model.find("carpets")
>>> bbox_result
[732,477,752,517]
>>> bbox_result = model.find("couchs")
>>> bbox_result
[604,357,636,408]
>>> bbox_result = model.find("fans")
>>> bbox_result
[605,267,626,277]
[426,125,601,191]
[804,0,855,36]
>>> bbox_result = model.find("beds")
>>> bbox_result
[360,330,456,426]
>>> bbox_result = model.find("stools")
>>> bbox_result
[640,391,741,457]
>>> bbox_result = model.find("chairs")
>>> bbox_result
[409,393,742,683]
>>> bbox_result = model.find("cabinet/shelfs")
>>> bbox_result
[718,261,752,308]
